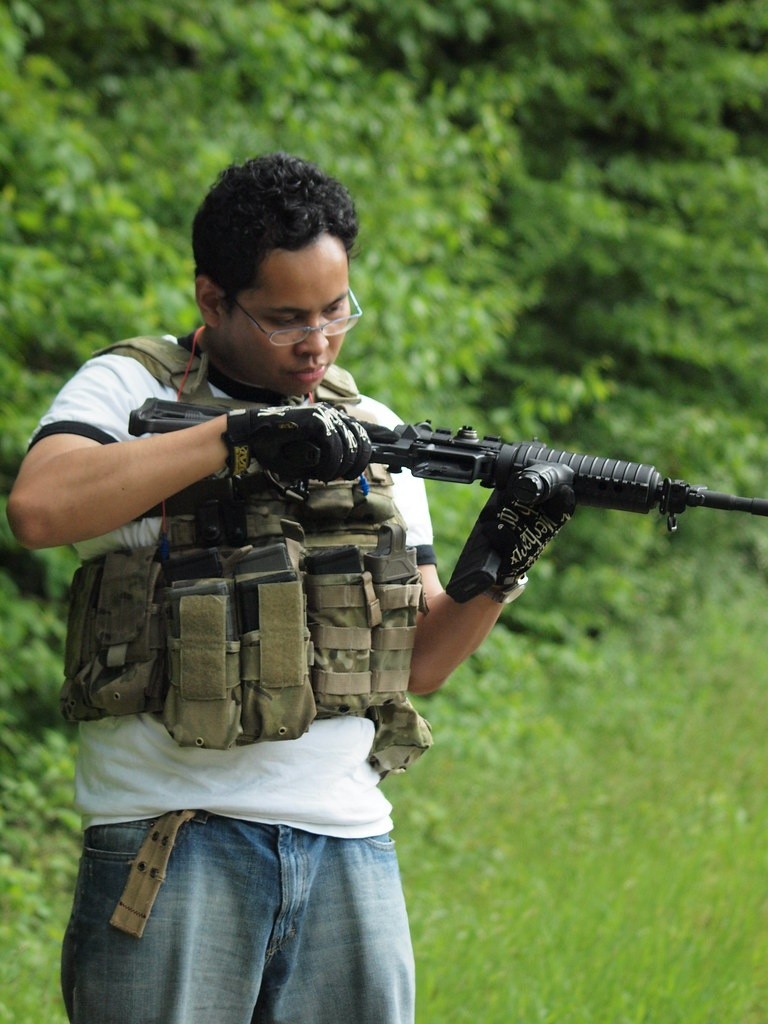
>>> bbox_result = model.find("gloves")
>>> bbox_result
[479,484,577,586]
[221,401,371,483]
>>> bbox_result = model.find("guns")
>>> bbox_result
[126,395,767,536]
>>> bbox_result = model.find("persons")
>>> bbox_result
[6,152,575,1024]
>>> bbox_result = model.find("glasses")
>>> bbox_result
[226,287,362,347]
